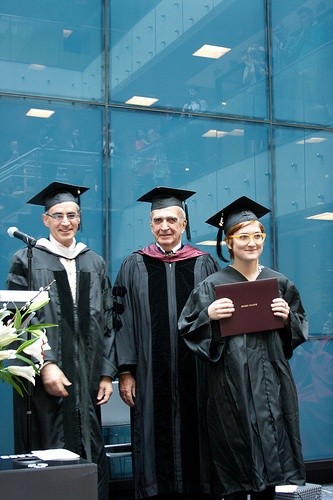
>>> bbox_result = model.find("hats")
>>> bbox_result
[205,196,271,262]
[26,182,91,233]
[137,186,196,242]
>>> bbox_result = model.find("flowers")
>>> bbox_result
[0,277,59,397]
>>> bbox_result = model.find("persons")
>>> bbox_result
[0,85,209,208]
[7,181,118,500]
[179,196,307,500]
[241,6,333,157]
[113,185,221,500]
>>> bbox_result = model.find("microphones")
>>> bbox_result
[6,227,37,246]
